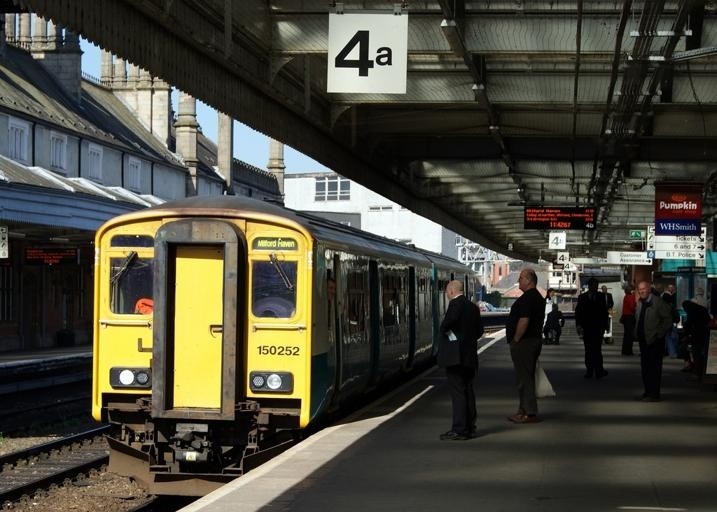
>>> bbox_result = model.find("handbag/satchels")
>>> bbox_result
[535,359,556,399]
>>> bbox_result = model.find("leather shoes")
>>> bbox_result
[508,413,536,423]
[635,393,660,402]
[584,368,608,379]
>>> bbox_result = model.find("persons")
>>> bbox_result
[440,279,484,440]
[327,278,336,300]
[506,269,546,423]
[542,278,713,403]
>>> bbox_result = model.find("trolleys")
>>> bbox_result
[602,314,614,344]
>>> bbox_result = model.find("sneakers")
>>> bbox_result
[440,430,476,441]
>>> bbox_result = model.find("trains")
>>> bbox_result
[92,194,480,497]
